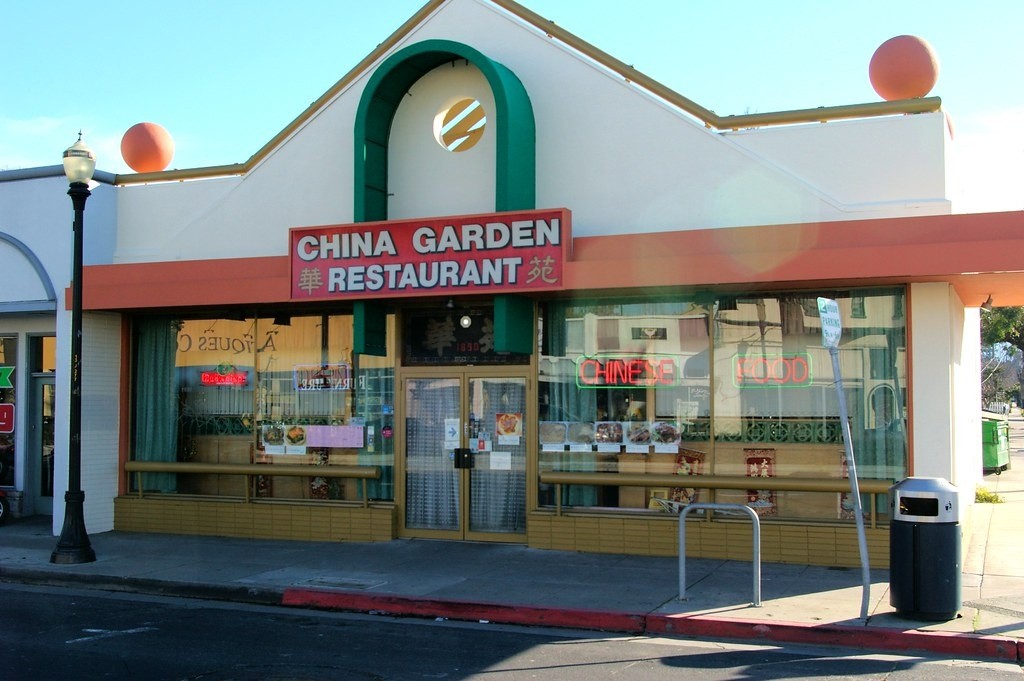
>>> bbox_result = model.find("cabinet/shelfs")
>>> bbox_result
[177,436,359,501]
[618,442,846,519]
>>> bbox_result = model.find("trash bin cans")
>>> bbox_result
[983,419,1010,475]
[887,476,961,621]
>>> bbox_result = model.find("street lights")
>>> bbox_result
[49,130,96,566]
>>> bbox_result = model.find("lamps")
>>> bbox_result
[458,306,472,328]
